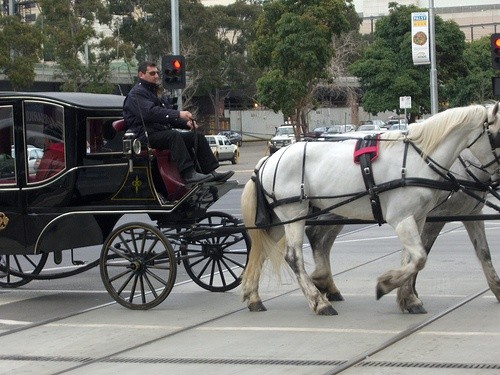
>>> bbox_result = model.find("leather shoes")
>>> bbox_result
[209,169,235,182]
[184,171,213,188]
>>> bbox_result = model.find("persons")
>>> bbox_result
[123,61,235,189]
[34,121,64,182]
[99,119,124,152]
[0,142,15,179]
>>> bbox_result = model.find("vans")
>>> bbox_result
[205,134,240,165]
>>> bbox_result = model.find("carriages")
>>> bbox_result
[0,92,500,317]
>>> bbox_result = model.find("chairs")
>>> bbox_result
[112,119,186,200]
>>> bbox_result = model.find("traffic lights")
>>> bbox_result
[159,53,187,89]
[492,76,500,97]
[490,32,500,70]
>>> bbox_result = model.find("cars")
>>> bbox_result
[312,119,409,142]
[8,142,47,180]
[217,131,242,147]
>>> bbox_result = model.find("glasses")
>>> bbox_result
[146,71,160,76]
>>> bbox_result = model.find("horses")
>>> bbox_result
[240,98,500,316]
[303,148,500,306]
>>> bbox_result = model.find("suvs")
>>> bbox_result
[268,125,305,154]
